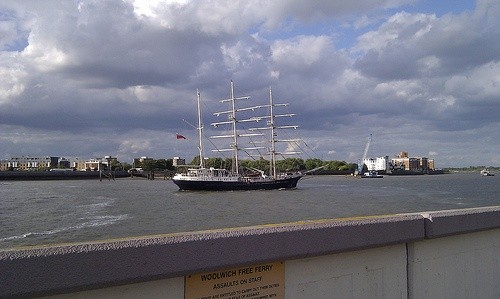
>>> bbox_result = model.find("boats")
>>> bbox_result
[480,167,496,177]
[171,78,330,198]
[361,171,383,178]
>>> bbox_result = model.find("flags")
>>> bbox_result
[176,134,186,139]
